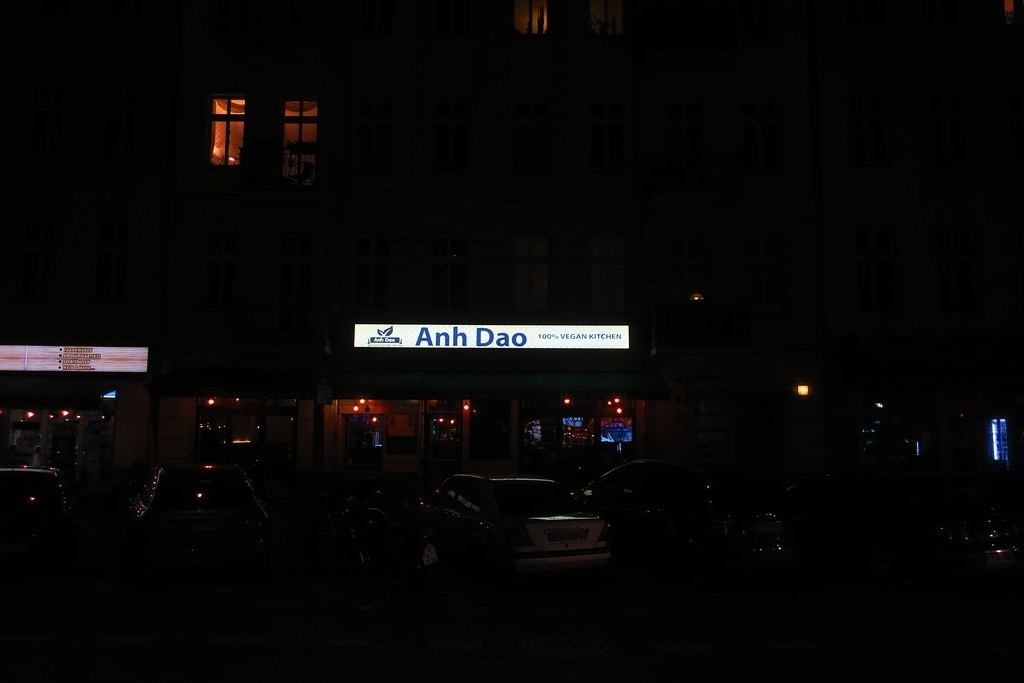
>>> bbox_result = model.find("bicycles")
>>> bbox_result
[286,481,408,594]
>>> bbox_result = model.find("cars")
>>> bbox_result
[418,473,613,585]
[0,467,79,564]
[129,463,272,576]
[787,472,1024,581]
[576,458,809,584]
